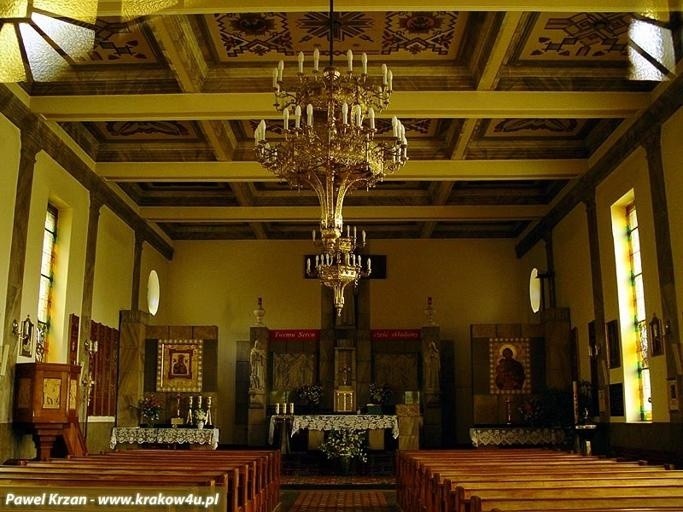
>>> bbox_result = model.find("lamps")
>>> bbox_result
[252,1,412,319]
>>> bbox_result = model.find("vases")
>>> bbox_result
[367,402,393,415]
[196,421,205,429]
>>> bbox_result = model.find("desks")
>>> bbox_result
[268,412,399,458]
[112,426,220,452]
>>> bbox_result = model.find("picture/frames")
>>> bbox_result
[489,337,534,395]
[18,321,35,357]
[156,339,206,394]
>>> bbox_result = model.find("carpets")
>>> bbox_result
[284,490,390,511]
[279,467,399,484]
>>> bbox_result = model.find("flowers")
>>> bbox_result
[192,408,207,421]
[138,392,162,421]
[365,382,391,405]
[293,384,324,407]
[316,426,369,464]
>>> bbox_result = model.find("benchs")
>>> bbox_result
[0,449,282,512]
[395,447,683,512]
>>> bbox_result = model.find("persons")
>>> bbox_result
[495,348,525,389]
[424,341,440,392]
[250,340,264,391]
[495,359,518,389]
